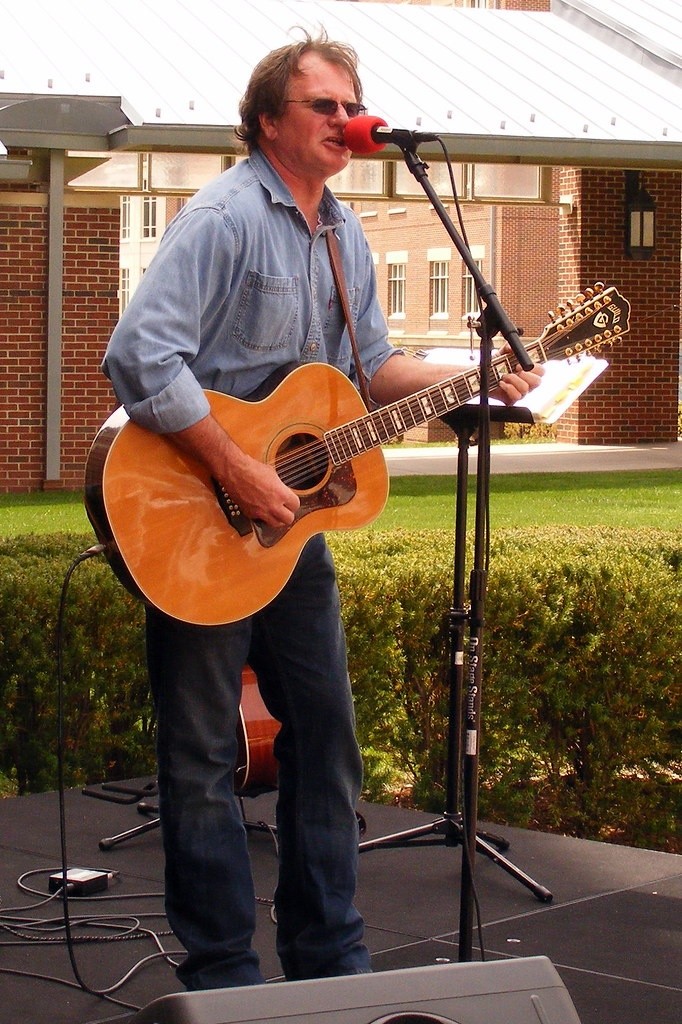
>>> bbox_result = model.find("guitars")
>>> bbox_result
[83,282,632,629]
[233,664,282,797]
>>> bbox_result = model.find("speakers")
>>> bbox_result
[130,955,582,1024]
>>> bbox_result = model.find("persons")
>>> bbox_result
[102,26,545,992]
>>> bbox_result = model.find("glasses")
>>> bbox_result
[284,97,367,118]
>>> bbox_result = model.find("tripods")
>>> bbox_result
[101,802,279,850]
[359,347,609,903]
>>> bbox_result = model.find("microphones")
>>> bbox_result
[342,116,439,155]
